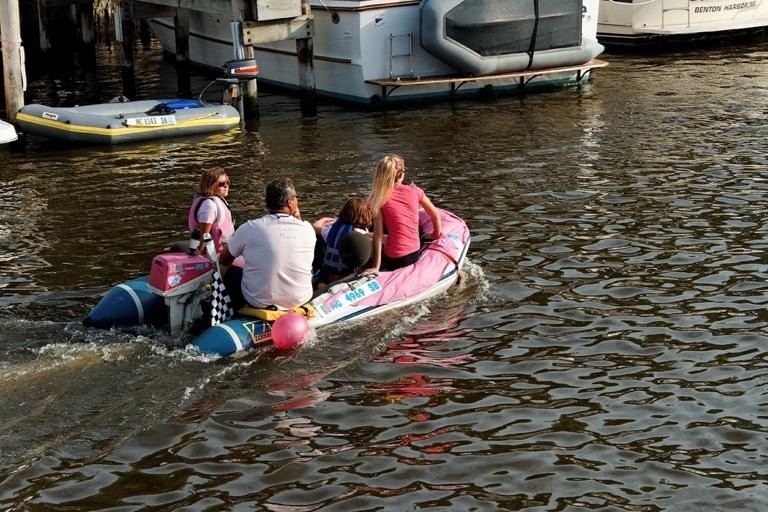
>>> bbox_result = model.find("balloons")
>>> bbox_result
[270,312,310,349]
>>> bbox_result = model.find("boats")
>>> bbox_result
[15,97,242,147]
[81,204,472,365]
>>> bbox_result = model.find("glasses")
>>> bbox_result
[217,180,231,188]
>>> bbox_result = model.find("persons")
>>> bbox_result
[189,166,245,269]
[313,196,373,291]
[358,153,442,276]
[186,177,317,336]
